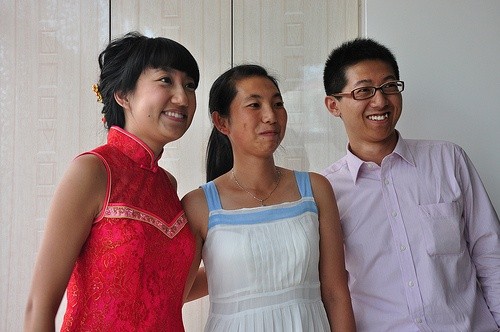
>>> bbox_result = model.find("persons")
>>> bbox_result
[24,32,208,332]
[181,65,356,331]
[319,37,500,332]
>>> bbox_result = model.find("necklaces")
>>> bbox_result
[230,169,281,207]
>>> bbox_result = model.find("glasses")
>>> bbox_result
[330,80,405,101]
